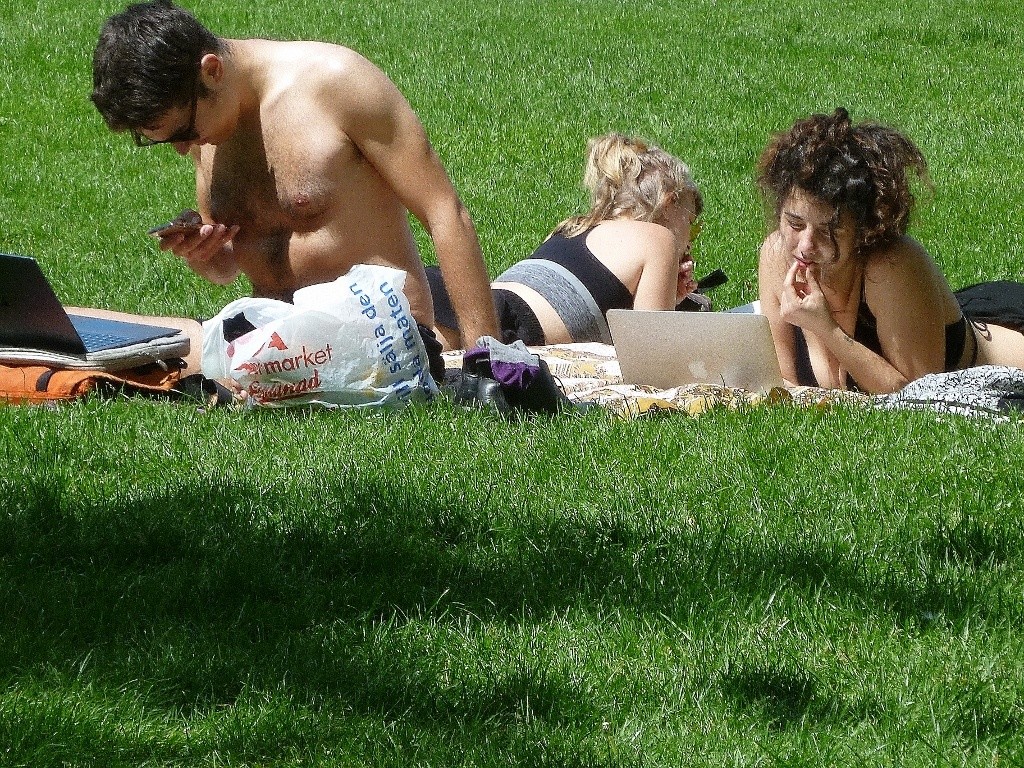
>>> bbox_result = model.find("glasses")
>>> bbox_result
[134,90,197,148]
[677,202,703,240]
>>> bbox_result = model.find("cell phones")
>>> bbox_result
[147,221,230,239]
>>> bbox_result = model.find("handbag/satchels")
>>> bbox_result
[199,262,437,410]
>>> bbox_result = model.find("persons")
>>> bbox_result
[756,108,1024,394]
[424,131,704,354]
[61,0,501,390]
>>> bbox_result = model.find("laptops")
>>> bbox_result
[605,309,809,398]
[0,253,183,354]
